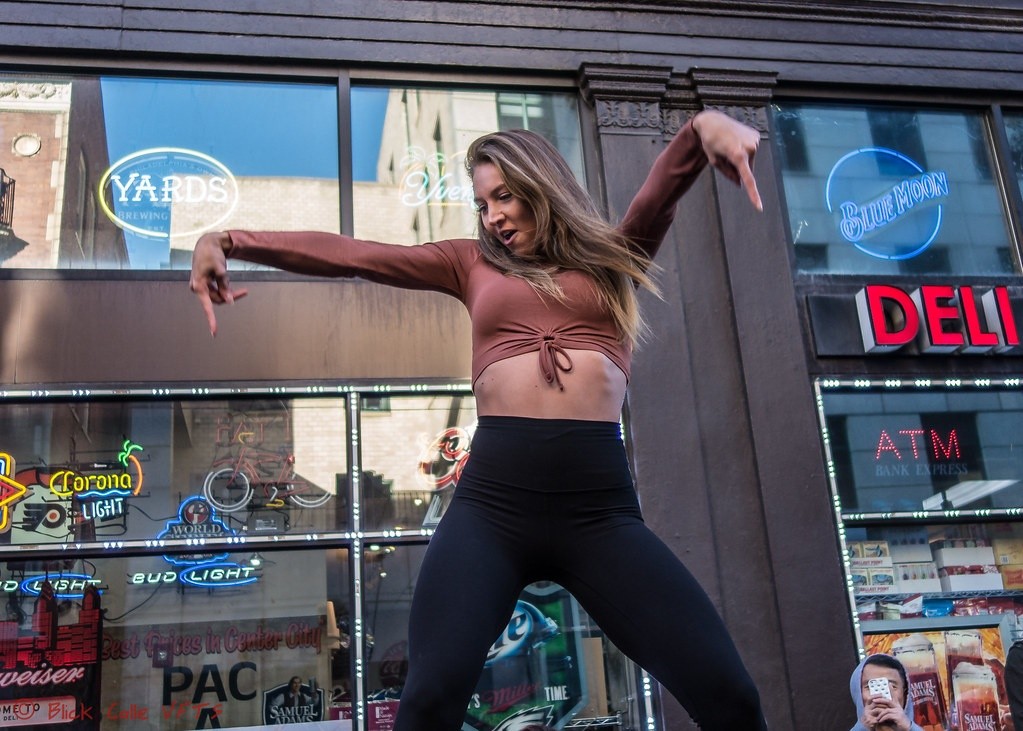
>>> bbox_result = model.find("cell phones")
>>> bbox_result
[867,677,892,701]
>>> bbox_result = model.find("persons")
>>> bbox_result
[849,653,924,731]
[189,112,767,731]
[1003,640,1023,731]
